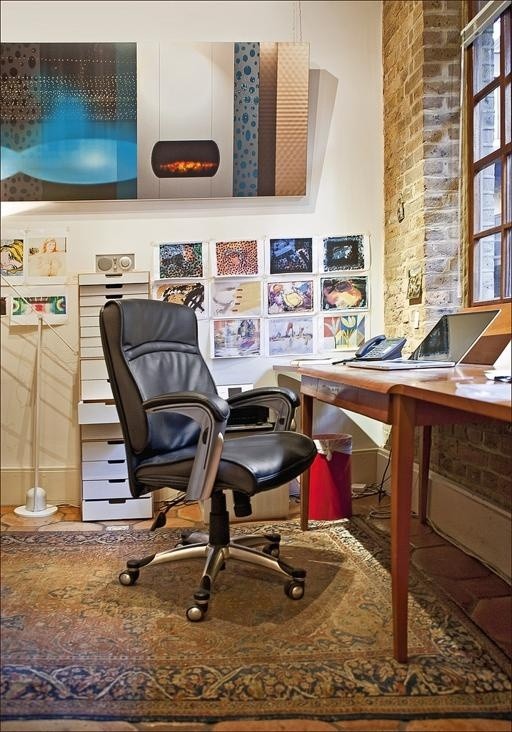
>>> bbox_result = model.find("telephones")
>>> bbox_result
[355,335,407,361]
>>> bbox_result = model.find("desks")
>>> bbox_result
[296,355,512,664]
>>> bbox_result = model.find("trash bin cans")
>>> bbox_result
[309,434,353,522]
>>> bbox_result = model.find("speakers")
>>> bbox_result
[96,254,135,272]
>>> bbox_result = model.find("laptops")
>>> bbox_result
[347,309,502,369]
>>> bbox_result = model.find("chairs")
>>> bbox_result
[99,299,317,622]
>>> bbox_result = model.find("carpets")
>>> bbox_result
[0,515,512,732]
